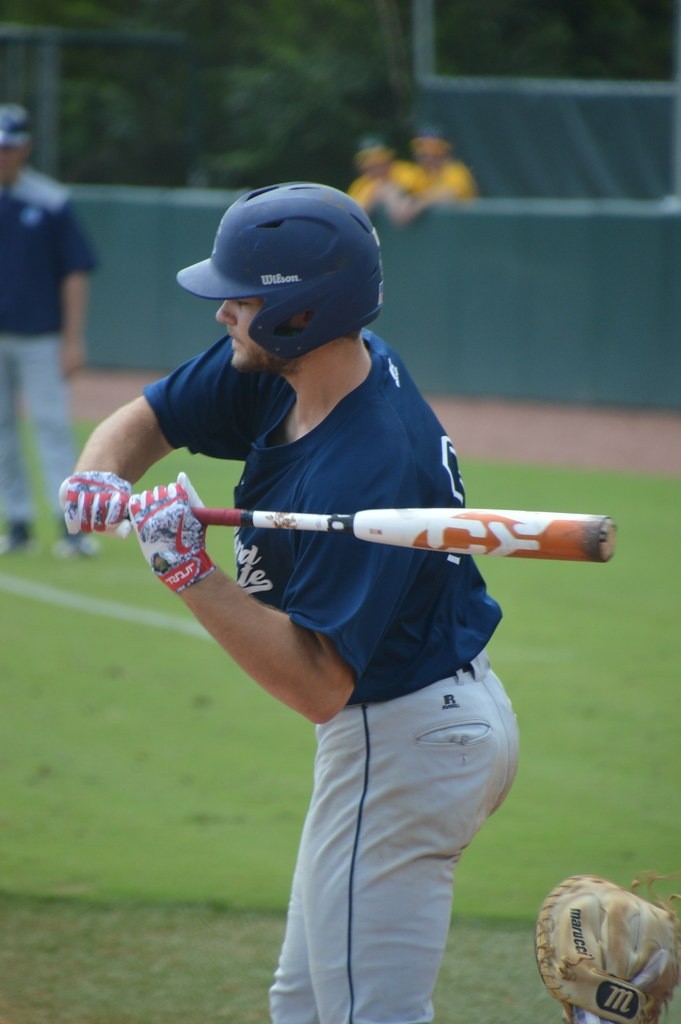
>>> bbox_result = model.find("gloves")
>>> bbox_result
[128,472,215,594]
[59,470,134,539]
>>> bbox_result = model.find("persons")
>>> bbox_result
[59,190,519,1024]
[0,103,99,559]
[348,123,472,225]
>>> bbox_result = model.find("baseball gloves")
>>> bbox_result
[536,869,680,1023]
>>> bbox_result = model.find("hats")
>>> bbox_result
[0,103,31,147]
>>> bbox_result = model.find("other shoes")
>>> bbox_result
[0,523,28,555]
[60,530,90,560]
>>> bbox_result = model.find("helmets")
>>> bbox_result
[176,181,384,361]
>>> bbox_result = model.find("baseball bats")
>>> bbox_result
[120,501,617,567]
